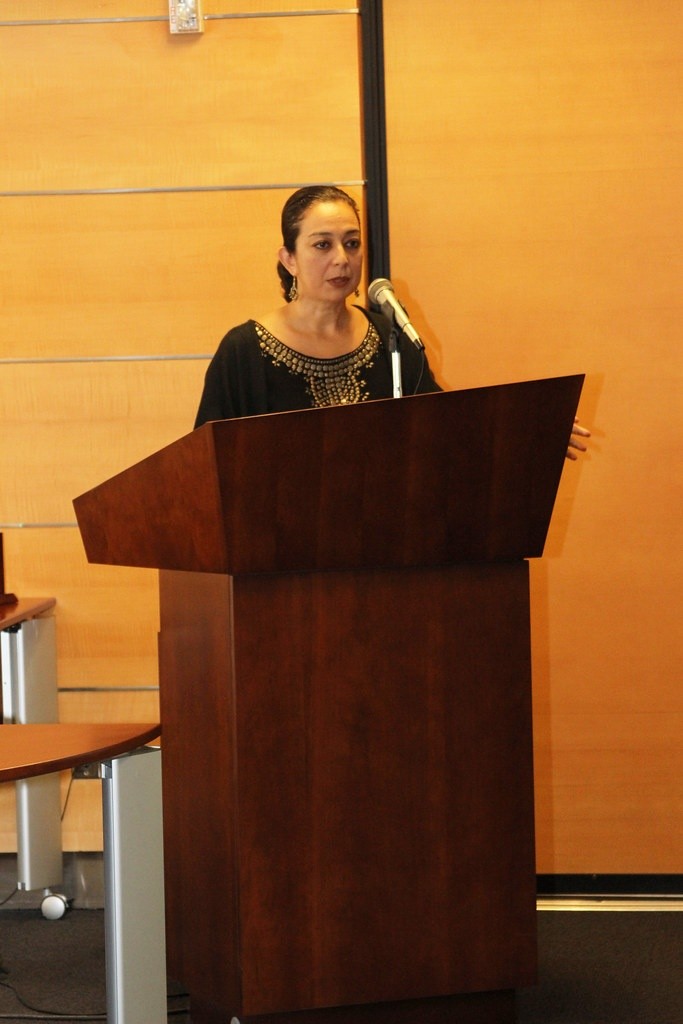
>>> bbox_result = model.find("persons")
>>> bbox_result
[194,185,591,463]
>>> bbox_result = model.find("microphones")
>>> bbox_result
[369,279,425,352]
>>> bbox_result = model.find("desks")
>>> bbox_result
[0,723,167,1024]
[0,598,61,892]
